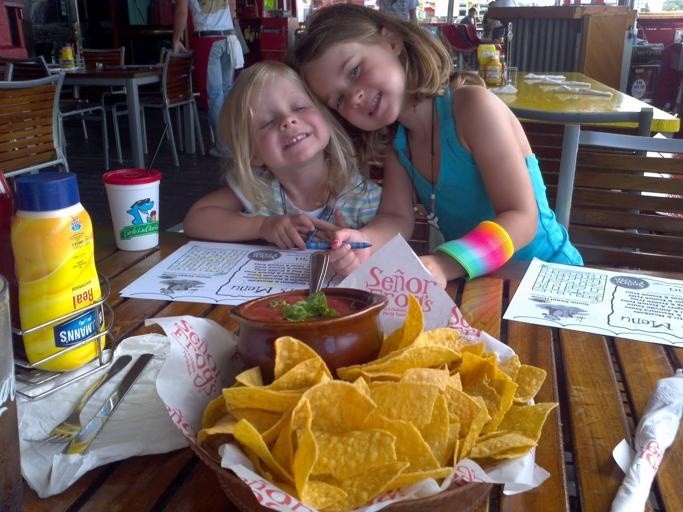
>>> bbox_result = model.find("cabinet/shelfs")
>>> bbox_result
[239,18,298,67]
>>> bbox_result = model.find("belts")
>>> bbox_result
[193,29,236,36]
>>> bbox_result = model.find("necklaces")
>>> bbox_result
[404,99,440,231]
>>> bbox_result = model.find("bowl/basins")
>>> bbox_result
[228,289,391,386]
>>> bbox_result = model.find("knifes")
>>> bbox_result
[46,353,154,482]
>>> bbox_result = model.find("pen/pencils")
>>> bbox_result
[306,207,333,246]
[307,242,373,249]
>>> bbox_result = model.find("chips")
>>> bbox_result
[197,293,560,512]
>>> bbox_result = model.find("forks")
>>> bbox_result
[25,354,130,445]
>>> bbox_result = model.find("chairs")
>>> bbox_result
[503,108,660,269]
[0,45,207,191]
[555,118,682,275]
[439,23,508,71]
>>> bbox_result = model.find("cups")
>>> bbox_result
[100,168,163,251]
[0,272,24,511]
[503,67,517,89]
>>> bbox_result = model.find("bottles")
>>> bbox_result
[476,37,507,88]
[9,171,105,372]
[57,42,75,71]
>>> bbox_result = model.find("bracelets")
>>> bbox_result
[433,220,515,281]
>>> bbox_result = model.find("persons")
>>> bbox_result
[375,0,517,57]
[288,1,587,291]
[183,59,383,252]
[170,1,246,154]
